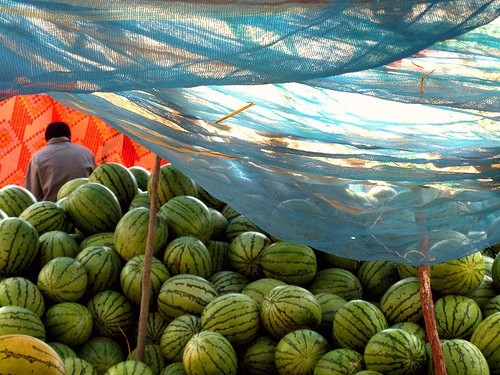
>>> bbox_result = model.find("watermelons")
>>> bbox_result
[0,164,500,375]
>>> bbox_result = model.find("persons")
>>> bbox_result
[25,122,97,202]
